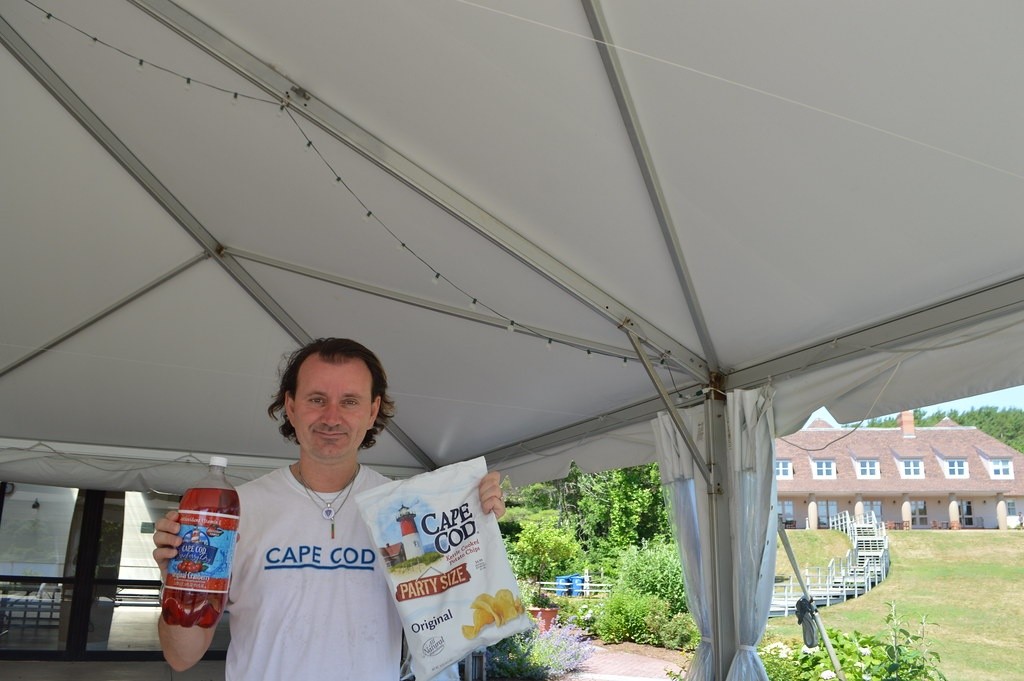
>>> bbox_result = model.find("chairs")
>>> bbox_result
[778,513,963,529]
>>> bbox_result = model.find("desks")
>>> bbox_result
[0,584,62,627]
[894,522,903,530]
[942,521,949,529]
[781,521,793,529]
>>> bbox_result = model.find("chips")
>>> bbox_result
[461,589,523,640]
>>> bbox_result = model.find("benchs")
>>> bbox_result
[0,606,60,628]
[0,597,60,620]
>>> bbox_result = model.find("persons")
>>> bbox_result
[153,337,505,681]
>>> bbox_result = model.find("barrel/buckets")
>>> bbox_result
[556,576,585,597]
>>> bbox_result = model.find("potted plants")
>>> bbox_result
[526,593,560,633]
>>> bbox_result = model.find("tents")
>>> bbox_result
[0,0,1024,681]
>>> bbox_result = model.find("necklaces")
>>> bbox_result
[298,459,363,539]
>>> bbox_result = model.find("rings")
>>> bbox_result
[500,490,504,501]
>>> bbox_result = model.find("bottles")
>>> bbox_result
[162,454,241,628]
